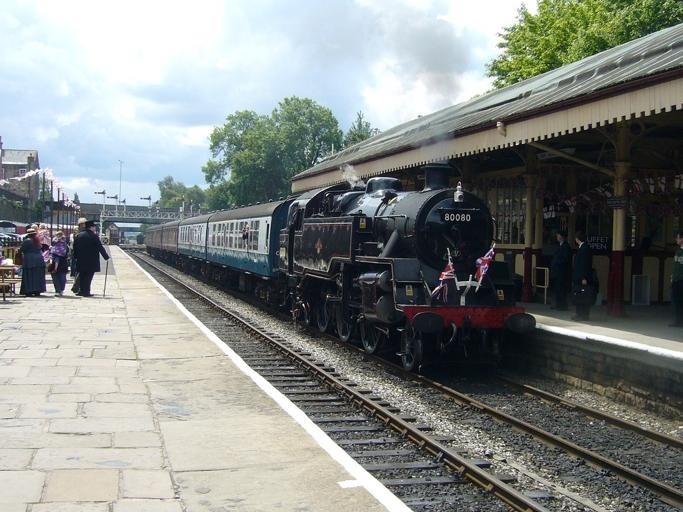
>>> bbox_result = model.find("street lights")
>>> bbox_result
[40,169,82,242]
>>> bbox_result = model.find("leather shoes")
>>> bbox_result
[71,287,94,296]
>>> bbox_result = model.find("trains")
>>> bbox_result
[0,219,79,237]
[144,163,536,374]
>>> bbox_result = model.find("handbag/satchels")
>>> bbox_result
[570,289,596,307]
[13,251,23,265]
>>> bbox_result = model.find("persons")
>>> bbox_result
[51,232,69,298]
[72,218,109,297]
[20,224,46,298]
[549,231,572,310]
[36,224,50,251]
[668,232,682,327]
[571,230,593,320]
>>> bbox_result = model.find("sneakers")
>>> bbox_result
[571,313,589,321]
[26,293,40,297]
[54,291,64,296]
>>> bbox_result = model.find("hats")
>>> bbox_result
[26,223,64,238]
[74,217,97,230]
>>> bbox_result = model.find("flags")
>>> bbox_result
[437,257,457,300]
[474,249,494,280]
